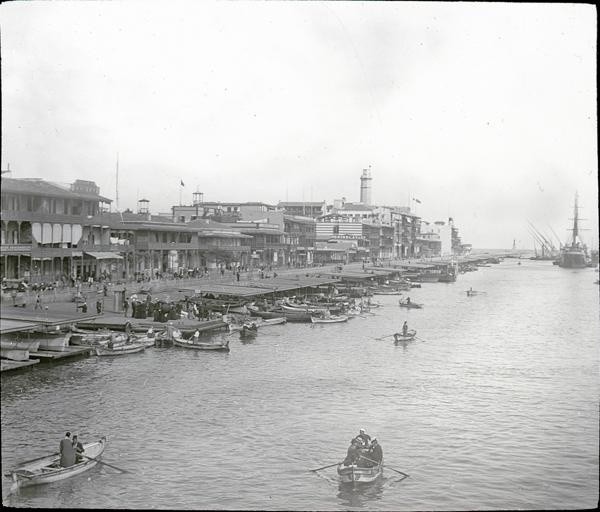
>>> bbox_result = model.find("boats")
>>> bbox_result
[172,336,230,351]
[240,326,256,337]
[394,331,416,340]
[438,276,456,282]
[95,335,157,356]
[10,438,105,488]
[250,278,422,323]
[338,460,382,484]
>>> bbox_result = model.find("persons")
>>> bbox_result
[403,320,408,336]
[77,302,87,312]
[355,429,372,448]
[71,436,85,465]
[406,296,411,304]
[34,294,43,311]
[58,432,75,468]
[193,328,200,343]
[132,298,137,317]
[344,439,361,466]
[359,445,378,469]
[370,438,383,462]
[123,297,129,319]
[96,301,102,313]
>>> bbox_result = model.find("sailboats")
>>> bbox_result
[558,194,591,268]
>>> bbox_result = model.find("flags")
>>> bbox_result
[416,200,421,204]
[181,182,184,187]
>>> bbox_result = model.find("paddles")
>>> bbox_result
[360,456,410,477]
